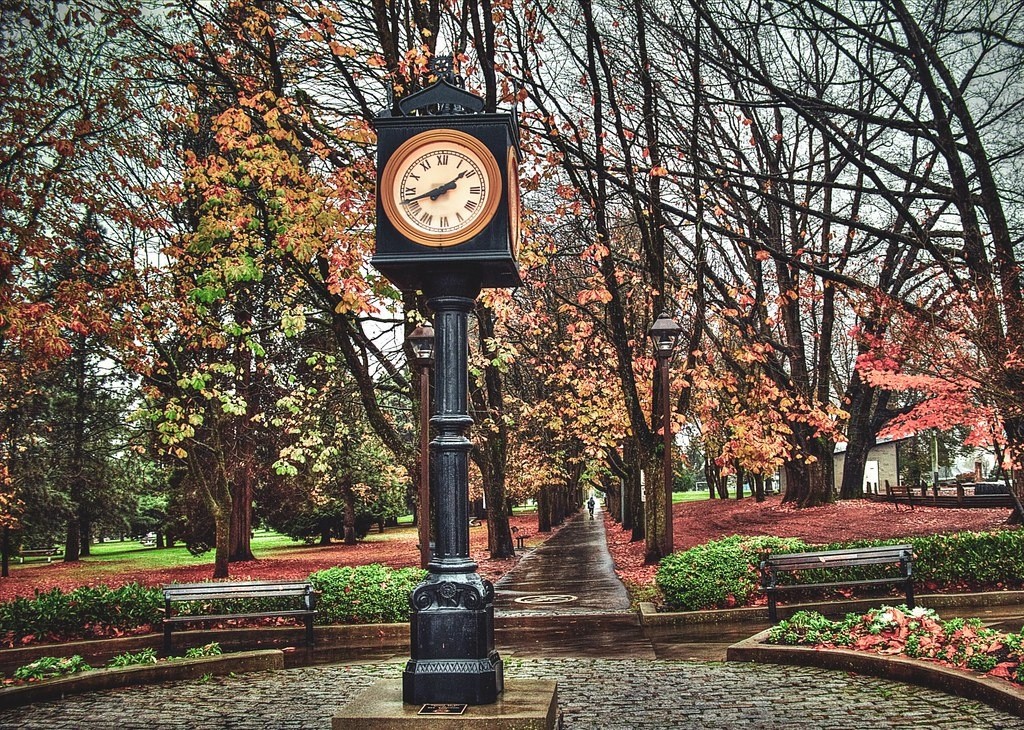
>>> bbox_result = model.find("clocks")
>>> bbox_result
[381,129,502,246]
[508,144,520,260]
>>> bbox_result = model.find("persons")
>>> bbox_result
[587,497,595,516]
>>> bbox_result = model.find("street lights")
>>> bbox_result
[646,312,684,557]
[404,318,434,567]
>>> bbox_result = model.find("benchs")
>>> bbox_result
[161,578,328,659]
[18,546,65,563]
[512,526,531,549]
[756,544,919,623]
